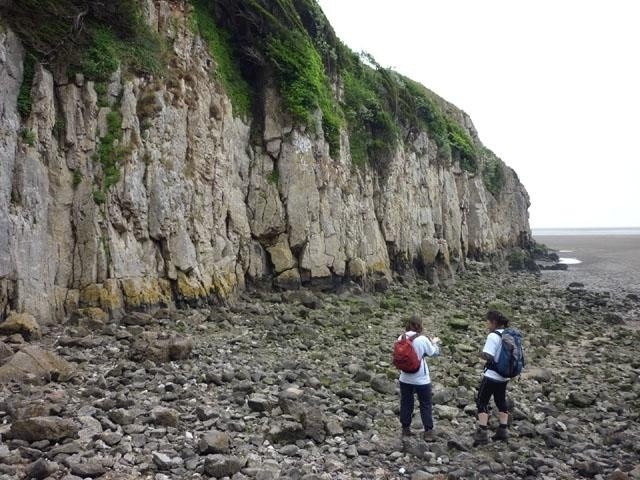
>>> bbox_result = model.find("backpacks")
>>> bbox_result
[392,333,426,374]
[484,327,524,378]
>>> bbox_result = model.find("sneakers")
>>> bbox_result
[497,423,510,433]
[478,425,489,431]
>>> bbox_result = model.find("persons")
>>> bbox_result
[394,315,442,442]
[473,310,525,442]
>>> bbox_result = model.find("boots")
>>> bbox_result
[424,429,436,441]
[402,426,417,441]
